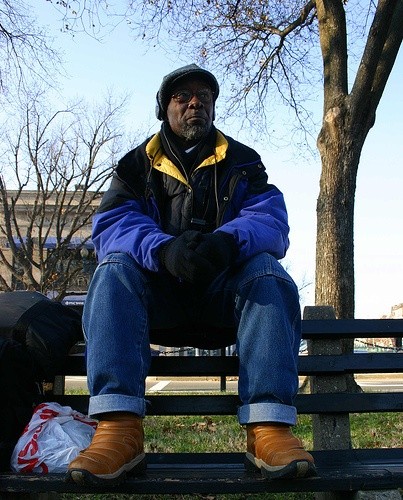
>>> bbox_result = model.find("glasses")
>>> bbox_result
[170,89,219,104]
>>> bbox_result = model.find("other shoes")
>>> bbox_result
[241,424,321,478]
[69,410,147,492]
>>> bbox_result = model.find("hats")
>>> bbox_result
[156,64,220,122]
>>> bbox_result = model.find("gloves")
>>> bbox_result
[162,229,239,290]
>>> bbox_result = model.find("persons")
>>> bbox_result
[67,63,318,486]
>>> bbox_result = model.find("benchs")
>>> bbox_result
[0,306,403,499]
